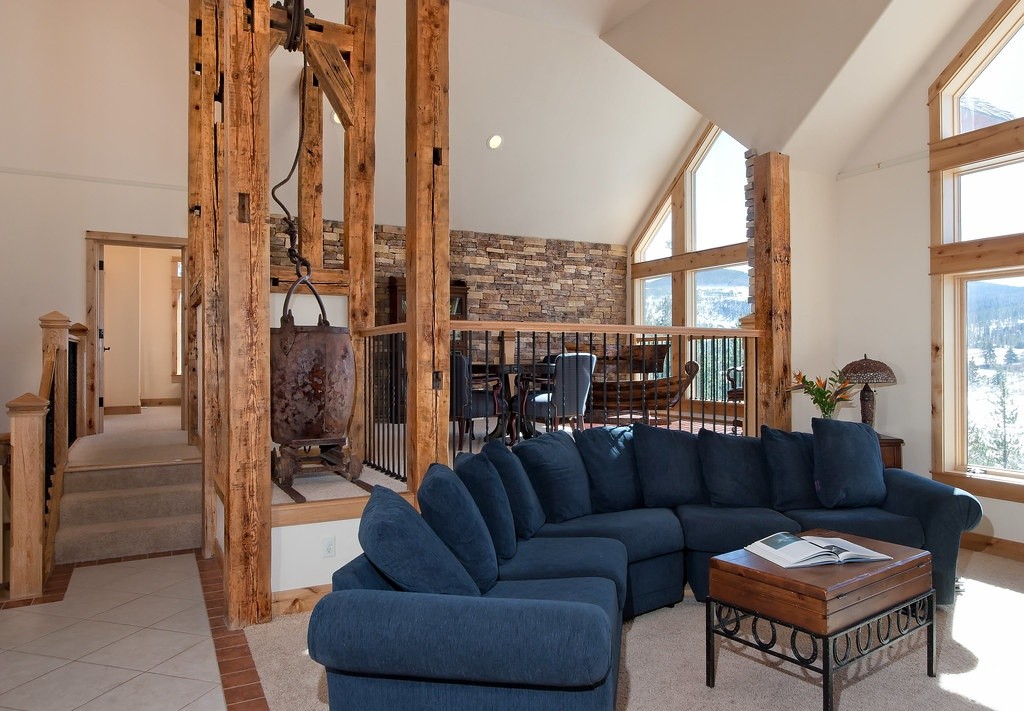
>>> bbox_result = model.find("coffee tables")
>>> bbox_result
[706,527,936,711]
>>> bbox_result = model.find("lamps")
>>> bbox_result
[838,354,897,429]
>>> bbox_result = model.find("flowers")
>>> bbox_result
[784,369,858,419]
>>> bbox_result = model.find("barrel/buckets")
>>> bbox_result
[270,258,356,450]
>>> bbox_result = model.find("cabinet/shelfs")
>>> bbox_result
[388,276,470,424]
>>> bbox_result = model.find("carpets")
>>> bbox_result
[244,547,1024,710]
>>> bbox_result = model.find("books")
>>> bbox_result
[744,532,893,569]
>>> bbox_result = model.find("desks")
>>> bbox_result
[468,362,555,443]
[878,434,904,468]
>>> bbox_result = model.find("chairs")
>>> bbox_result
[451,351,509,451]
[507,352,596,446]
[727,368,744,434]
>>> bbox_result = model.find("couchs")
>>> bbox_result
[308,468,983,711]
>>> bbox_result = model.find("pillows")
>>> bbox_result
[633,421,710,507]
[358,485,481,597]
[418,463,498,594]
[572,425,643,513]
[512,430,592,523]
[697,428,771,508]
[761,425,823,511]
[455,451,516,564]
[481,438,547,540]
[811,417,887,510]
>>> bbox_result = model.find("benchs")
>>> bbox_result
[565,344,699,424]
